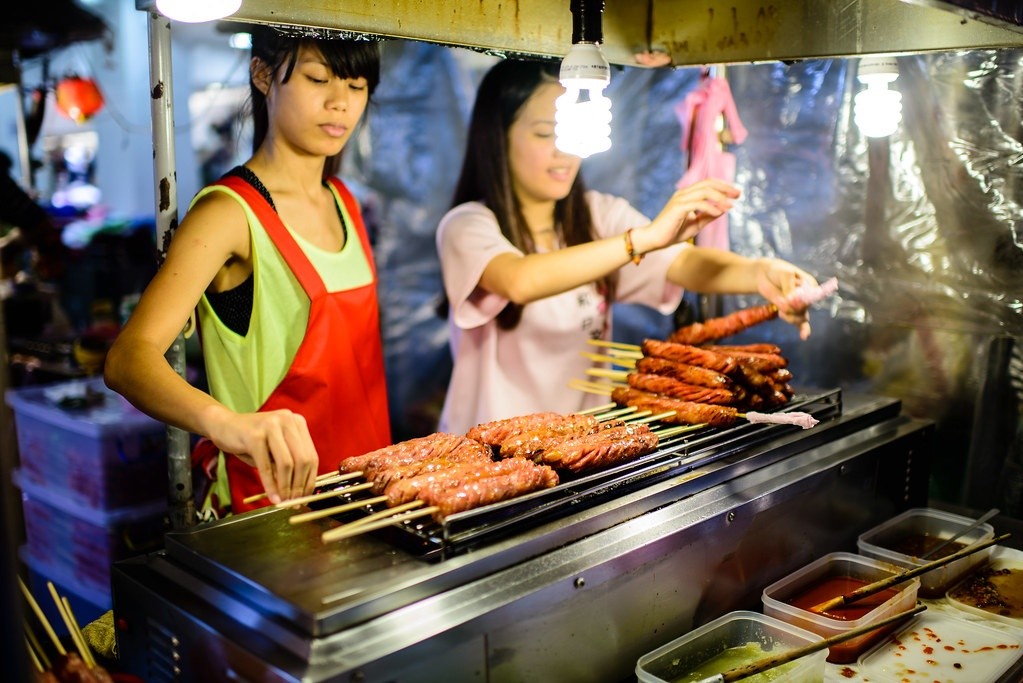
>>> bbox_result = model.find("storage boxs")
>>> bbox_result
[4,367,208,658]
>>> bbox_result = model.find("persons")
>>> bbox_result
[104,19,392,527]
[437,53,820,438]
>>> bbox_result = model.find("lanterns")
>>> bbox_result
[54,77,101,121]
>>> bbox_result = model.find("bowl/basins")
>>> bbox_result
[762,551,920,662]
[857,508,995,593]
[635,610,830,683]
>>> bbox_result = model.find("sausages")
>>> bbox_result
[30,652,115,683]
[610,300,795,428]
[338,410,658,518]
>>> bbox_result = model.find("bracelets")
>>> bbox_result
[624,228,645,264]
